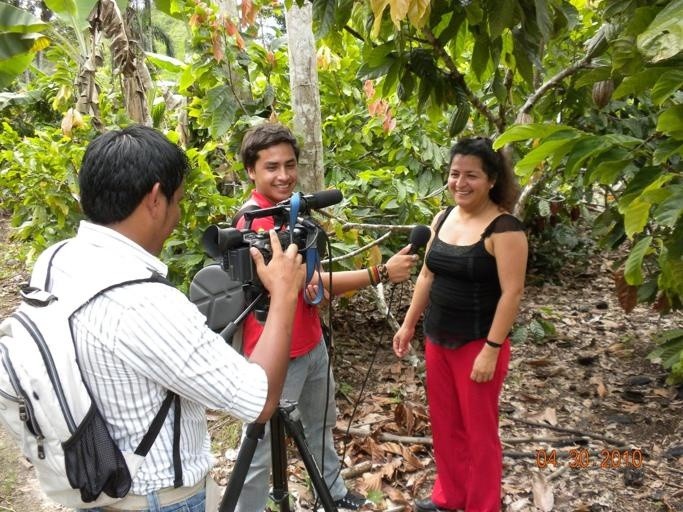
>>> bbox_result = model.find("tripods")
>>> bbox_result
[218,400,338,512]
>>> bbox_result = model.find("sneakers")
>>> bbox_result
[333,488,377,512]
[414,495,447,512]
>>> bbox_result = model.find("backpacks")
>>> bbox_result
[0,238,185,510]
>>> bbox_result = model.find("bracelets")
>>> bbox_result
[366,263,379,288]
[484,338,502,349]
[375,264,390,285]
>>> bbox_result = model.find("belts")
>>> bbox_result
[102,476,207,512]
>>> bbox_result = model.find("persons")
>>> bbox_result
[226,122,423,511]
[23,120,312,512]
[386,132,530,511]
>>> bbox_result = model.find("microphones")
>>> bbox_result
[407,226,431,257]
